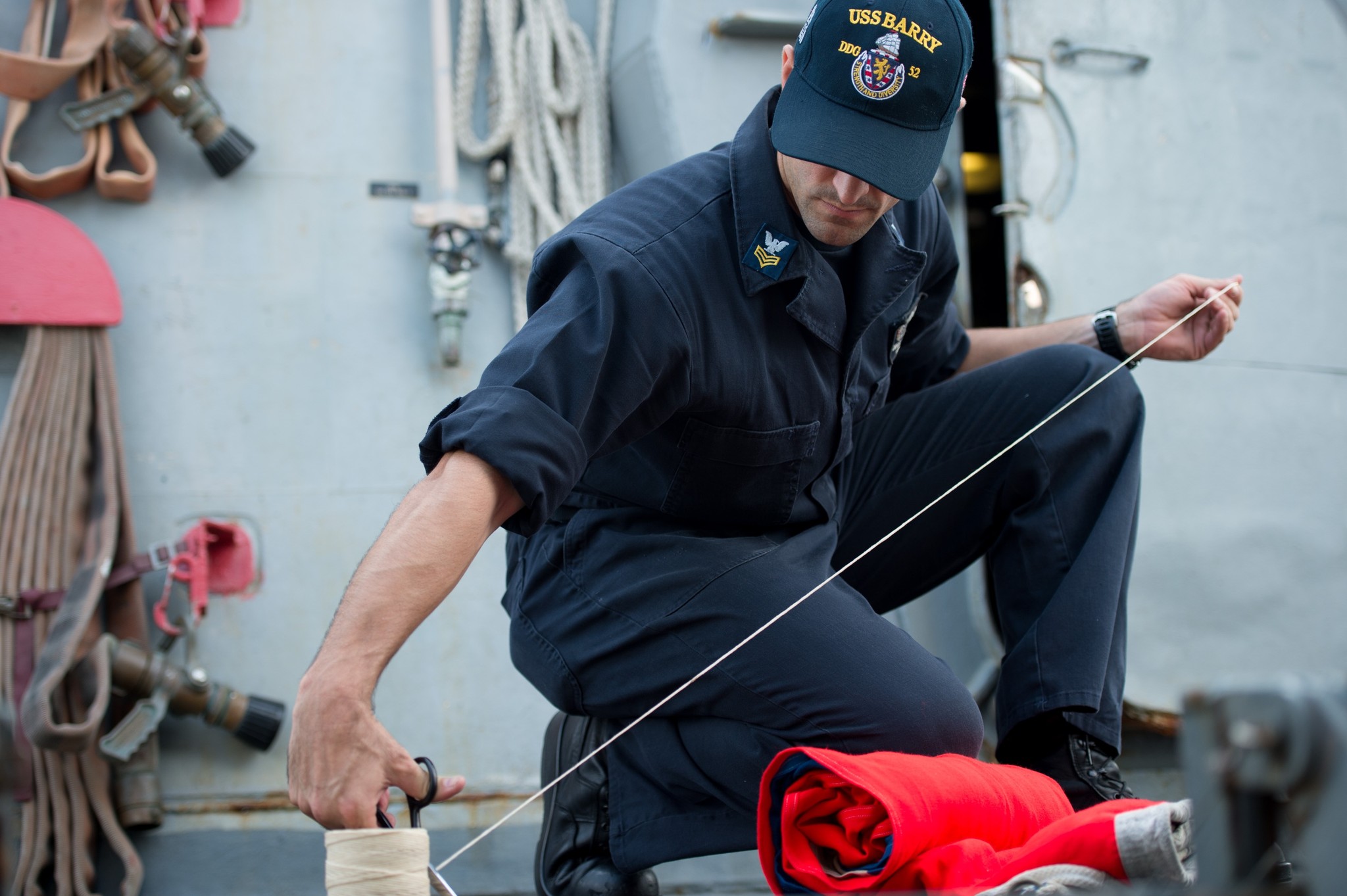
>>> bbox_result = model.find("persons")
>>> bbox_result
[284,0,1241,896]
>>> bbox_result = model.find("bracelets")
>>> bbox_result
[1092,307,1140,370]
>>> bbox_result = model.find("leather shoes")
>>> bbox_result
[1000,725,1139,810]
[535,710,661,894]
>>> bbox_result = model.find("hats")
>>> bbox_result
[771,0,974,202]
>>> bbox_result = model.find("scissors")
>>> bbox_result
[376,757,457,896]
